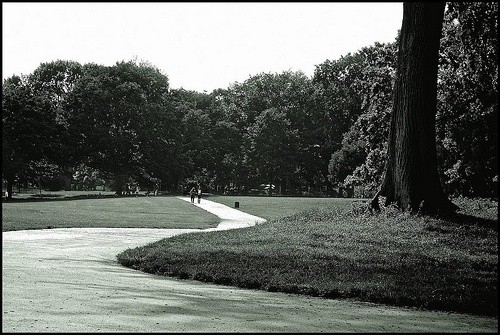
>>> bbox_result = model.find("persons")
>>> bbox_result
[189,187,196,204]
[197,187,203,204]
